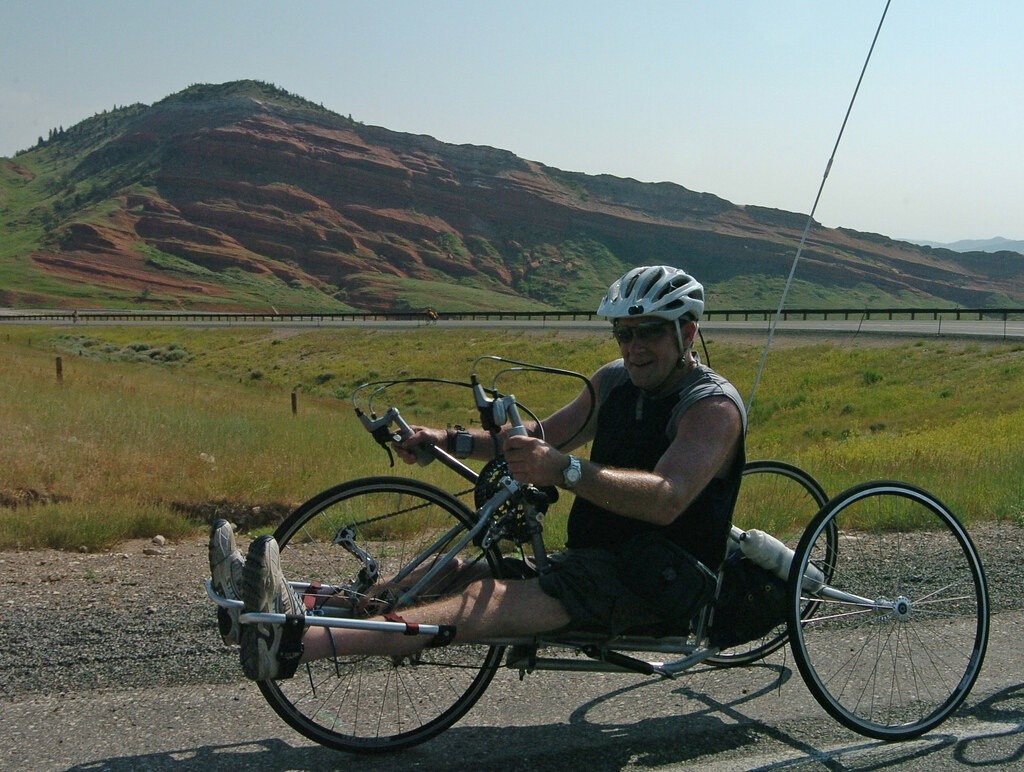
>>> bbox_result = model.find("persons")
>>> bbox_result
[209,267,747,681]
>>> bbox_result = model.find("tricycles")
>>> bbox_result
[202,354,992,758]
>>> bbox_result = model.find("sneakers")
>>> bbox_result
[209,519,244,644]
[240,535,308,680]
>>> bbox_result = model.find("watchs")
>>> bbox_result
[562,454,582,489]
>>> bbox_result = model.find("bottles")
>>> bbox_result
[740,529,824,594]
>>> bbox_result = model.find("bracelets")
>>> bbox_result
[446,422,473,460]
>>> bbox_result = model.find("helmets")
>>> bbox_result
[597,265,704,321]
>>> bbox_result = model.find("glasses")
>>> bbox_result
[614,321,685,343]
